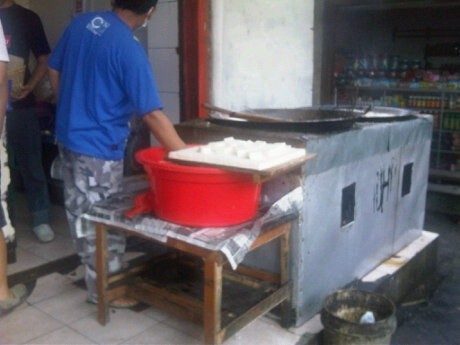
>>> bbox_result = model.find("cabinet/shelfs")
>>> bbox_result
[332,86,460,183]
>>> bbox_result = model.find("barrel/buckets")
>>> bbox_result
[320,290,397,345]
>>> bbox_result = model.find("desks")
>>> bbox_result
[80,189,298,345]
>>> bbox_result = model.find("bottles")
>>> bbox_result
[443,112,460,131]
[423,109,438,128]
[449,94,460,110]
[408,96,448,108]
[362,52,420,79]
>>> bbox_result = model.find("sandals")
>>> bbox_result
[86,296,140,308]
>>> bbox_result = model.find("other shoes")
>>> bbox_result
[33,223,54,242]
[0,283,27,315]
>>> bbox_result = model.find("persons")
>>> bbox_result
[0,0,54,310]
[47,0,189,308]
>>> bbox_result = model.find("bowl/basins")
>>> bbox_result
[136,144,261,227]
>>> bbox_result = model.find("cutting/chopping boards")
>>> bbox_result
[168,145,318,176]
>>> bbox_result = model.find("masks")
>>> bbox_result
[136,17,149,31]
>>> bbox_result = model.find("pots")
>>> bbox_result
[311,105,412,121]
[208,103,372,134]
